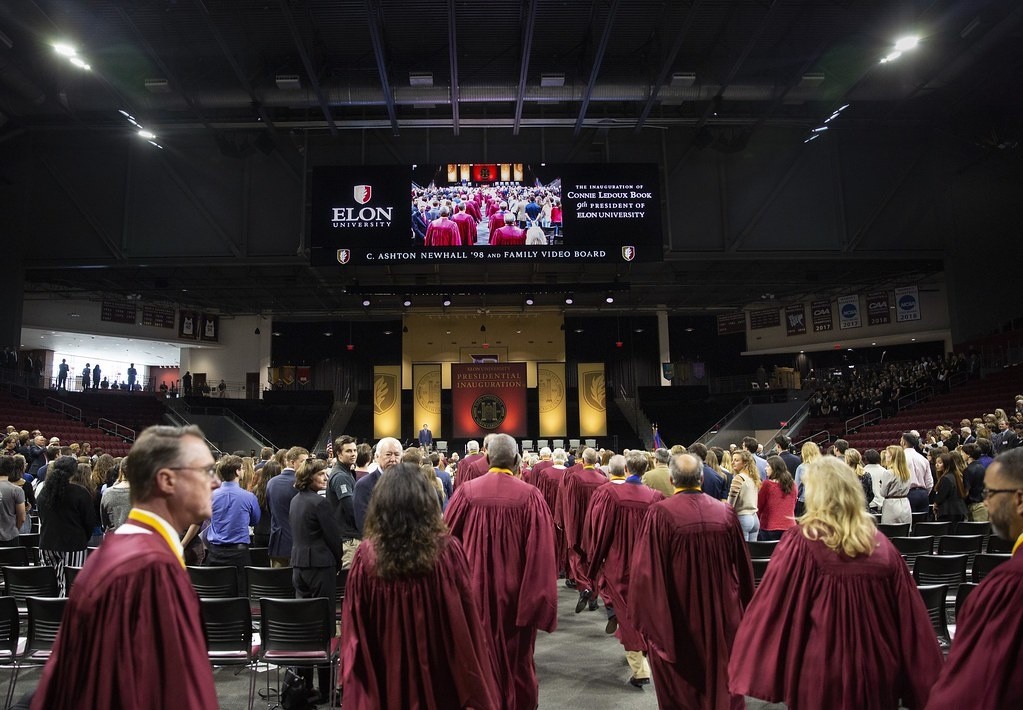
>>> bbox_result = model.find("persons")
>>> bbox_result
[808,345,980,418]
[82,363,90,389]
[111,380,118,389]
[0,390,1023,710]
[412,178,562,245]
[135,381,142,390]
[101,377,109,388]
[128,364,136,391]
[144,382,152,391]
[196,383,210,393]
[419,424,432,454]
[218,380,226,398]
[120,381,126,389]
[93,365,101,389]
[0,344,42,374]
[160,381,167,392]
[58,359,69,390]
[182,371,192,393]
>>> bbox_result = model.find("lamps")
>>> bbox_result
[561,317,565,330]
[481,322,485,331]
[255,316,260,334]
[403,326,408,332]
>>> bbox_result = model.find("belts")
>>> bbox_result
[210,543,249,549]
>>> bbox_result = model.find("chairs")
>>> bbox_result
[585,440,598,451]
[791,366,1023,464]
[746,496,1015,662]
[435,441,448,456]
[569,439,580,451]
[0,387,133,458]
[536,440,550,451]
[552,440,566,451]
[0,502,353,710]
[521,440,534,451]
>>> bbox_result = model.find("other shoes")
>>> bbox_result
[576,590,592,613]
[630,677,650,686]
[590,604,599,611]
[566,580,577,589]
[558,575,565,579]
[606,616,617,634]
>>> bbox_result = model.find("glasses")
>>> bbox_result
[153,464,217,478]
[980,487,1023,500]
